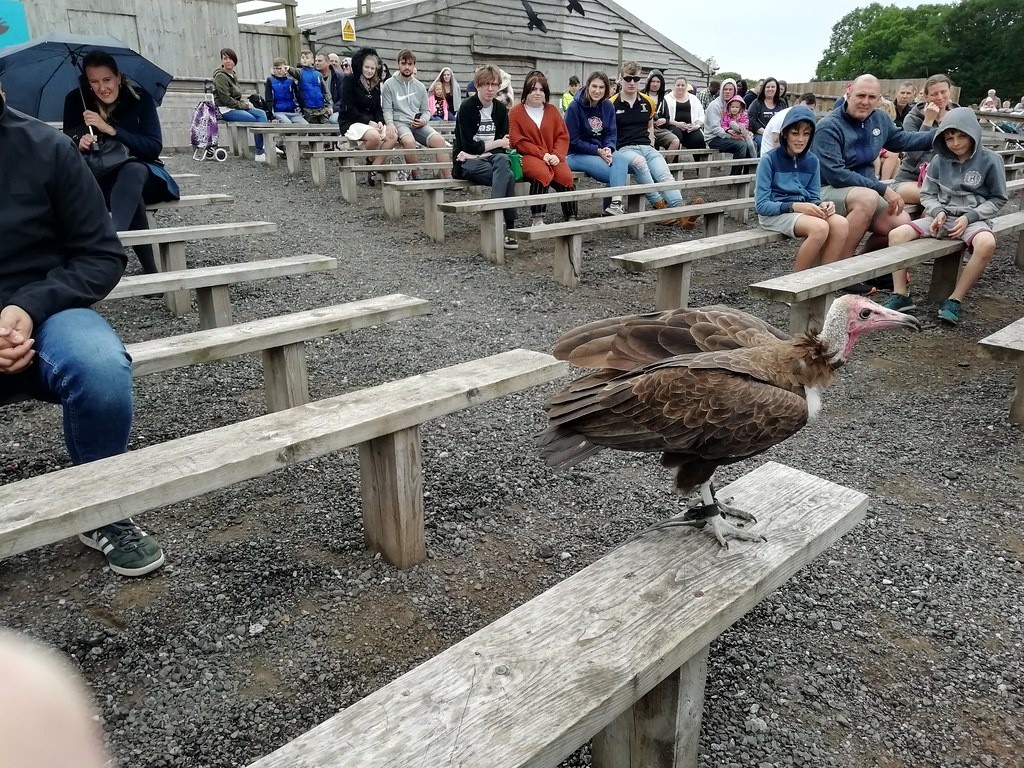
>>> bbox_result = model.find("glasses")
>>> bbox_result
[341,64,348,68]
[622,75,641,82]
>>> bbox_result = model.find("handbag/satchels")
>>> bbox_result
[80,137,136,178]
[506,149,524,181]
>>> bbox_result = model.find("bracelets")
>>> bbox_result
[476,153,479,158]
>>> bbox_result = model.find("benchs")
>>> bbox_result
[0,119,1024,768]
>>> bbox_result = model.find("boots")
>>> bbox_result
[679,197,705,229]
[652,199,678,225]
[130,227,163,297]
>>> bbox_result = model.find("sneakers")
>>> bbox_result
[880,292,916,313]
[78,517,164,577]
[836,282,876,295]
[937,298,962,324]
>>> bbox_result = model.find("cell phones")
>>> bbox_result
[414,113,422,122]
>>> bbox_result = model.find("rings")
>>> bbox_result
[92,119,93,121]
[85,136,88,140]
[91,115,93,119]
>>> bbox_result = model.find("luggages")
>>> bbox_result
[190,78,228,161]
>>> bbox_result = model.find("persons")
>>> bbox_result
[564,71,628,215]
[562,68,816,189]
[212,48,284,162]
[756,106,849,274]
[63,51,163,299]
[977,89,1024,123]
[265,50,393,159]
[508,70,578,226]
[0,77,165,576]
[383,49,464,190]
[608,60,704,228]
[0,629,112,768]
[810,74,962,297]
[451,63,519,250]
[338,47,398,187]
[412,66,514,142]
[882,107,1009,324]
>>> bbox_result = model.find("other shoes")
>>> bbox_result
[276,146,284,154]
[605,202,627,216]
[368,171,376,186]
[504,236,519,250]
[255,154,265,162]
[366,157,372,165]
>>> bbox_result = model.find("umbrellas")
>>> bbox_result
[0,30,174,155]
